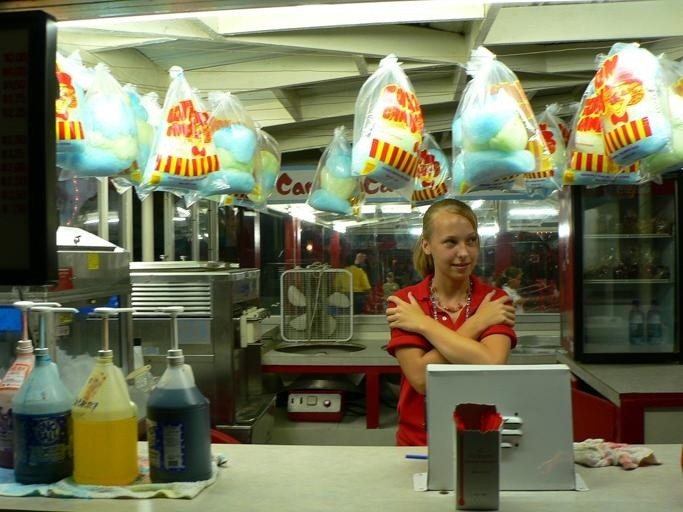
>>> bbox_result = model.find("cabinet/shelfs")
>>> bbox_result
[571,373,683,444]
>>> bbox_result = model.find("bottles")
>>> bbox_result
[628,299,663,347]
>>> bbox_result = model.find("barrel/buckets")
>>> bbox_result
[145,306,213,484]
[70,306,145,487]
[10,306,80,486]
[1,301,62,470]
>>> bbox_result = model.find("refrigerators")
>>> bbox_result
[557,183,682,364]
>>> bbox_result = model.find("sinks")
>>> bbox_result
[274,340,367,354]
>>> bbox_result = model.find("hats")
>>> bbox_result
[354,253,367,264]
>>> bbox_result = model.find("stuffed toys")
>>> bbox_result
[307,128,364,218]
[54,47,280,209]
[352,62,416,187]
[530,108,571,187]
[561,43,682,192]
[449,83,534,195]
[412,146,449,204]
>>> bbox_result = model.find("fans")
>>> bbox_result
[280,268,354,344]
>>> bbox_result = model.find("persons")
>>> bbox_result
[494,266,528,314]
[335,252,418,315]
[384,198,518,446]
